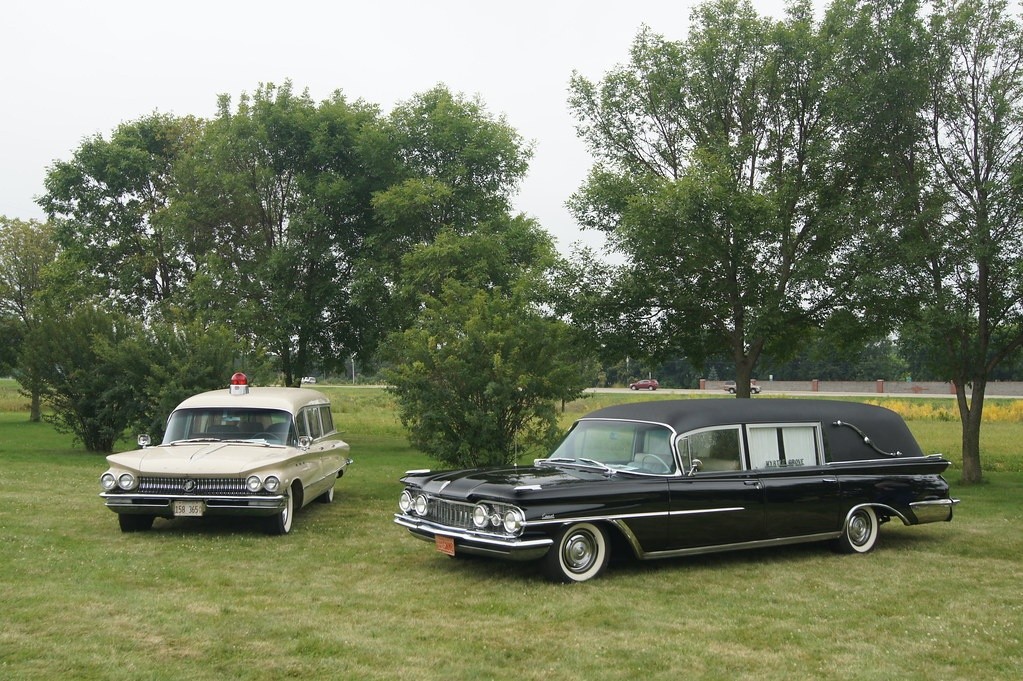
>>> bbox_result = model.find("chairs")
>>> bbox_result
[207,425,240,439]
[682,456,740,473]
[264,421,298,442]
[628,453,678,473]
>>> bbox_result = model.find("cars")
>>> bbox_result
[98,374,355,537]
[393,397,962,584]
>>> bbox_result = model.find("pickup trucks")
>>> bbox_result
[724,382,761,394]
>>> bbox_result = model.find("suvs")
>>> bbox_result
[629,379,659,391]
[301,376,316,385]
